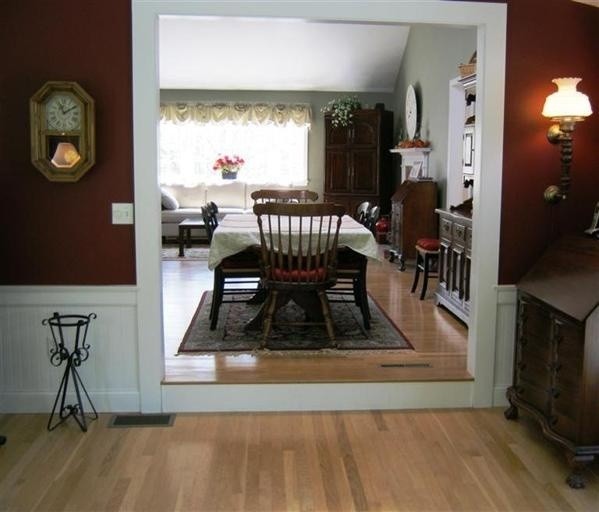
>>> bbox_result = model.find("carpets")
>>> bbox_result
[162,242,211,262]
[174,288,417,358]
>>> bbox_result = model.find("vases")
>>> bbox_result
[221,169,238,180]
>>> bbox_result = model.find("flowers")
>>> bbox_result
[212,154,246,177]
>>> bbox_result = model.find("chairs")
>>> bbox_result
[200,188,381,352]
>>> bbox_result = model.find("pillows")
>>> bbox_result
[160,186,179,211]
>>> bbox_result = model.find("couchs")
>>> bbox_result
[159,181,307,241]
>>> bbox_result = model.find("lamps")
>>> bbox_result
[541,76,595,206]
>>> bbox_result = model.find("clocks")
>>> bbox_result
[27,79,98,184]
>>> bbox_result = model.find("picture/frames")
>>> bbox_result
[409,161,423,181]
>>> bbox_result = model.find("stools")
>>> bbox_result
[410,238,441,301]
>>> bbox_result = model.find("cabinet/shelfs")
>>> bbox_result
[457,72,477,212]
[388,179,439,272]
[504,232,599,489]
[433,207,473,330]
[323,108,395,238]
